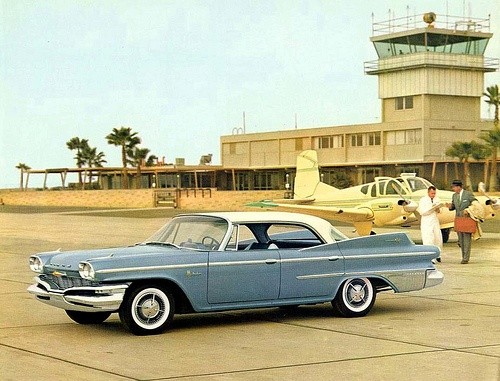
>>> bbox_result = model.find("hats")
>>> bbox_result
[451,179,463,186]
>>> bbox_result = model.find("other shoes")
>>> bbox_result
[432,257,441,264]
[461,260,469,264]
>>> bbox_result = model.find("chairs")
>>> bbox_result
[249,242,272,249]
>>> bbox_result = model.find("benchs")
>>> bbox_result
[268,240,321,250]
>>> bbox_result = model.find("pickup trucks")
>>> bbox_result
[27,211,443,336]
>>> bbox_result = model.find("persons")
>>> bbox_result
[419,186,443,264]
[445,180,479,264]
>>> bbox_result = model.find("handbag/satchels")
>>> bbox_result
[453,214,477,233]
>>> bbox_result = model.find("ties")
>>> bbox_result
[431,198,433,204]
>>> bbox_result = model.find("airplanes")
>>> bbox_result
[245,150,500,244]
